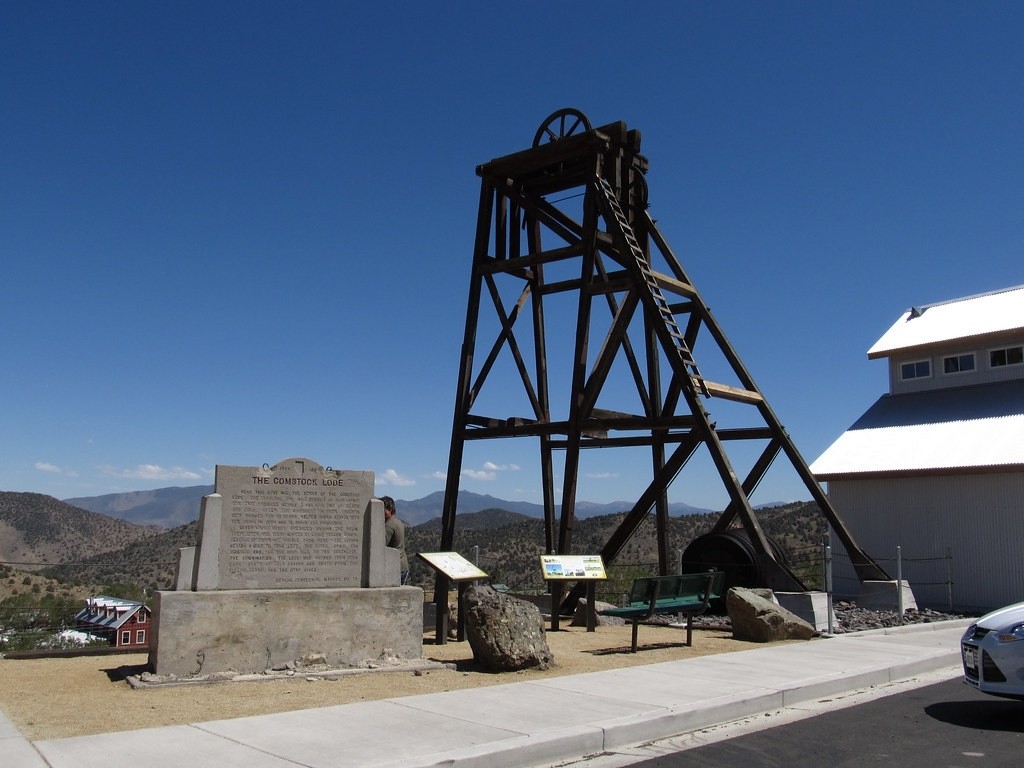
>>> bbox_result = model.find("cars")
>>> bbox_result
[0,610,50,647]
[961,599,1024,704]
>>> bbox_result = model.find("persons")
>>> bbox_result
[376,496,410,585]
